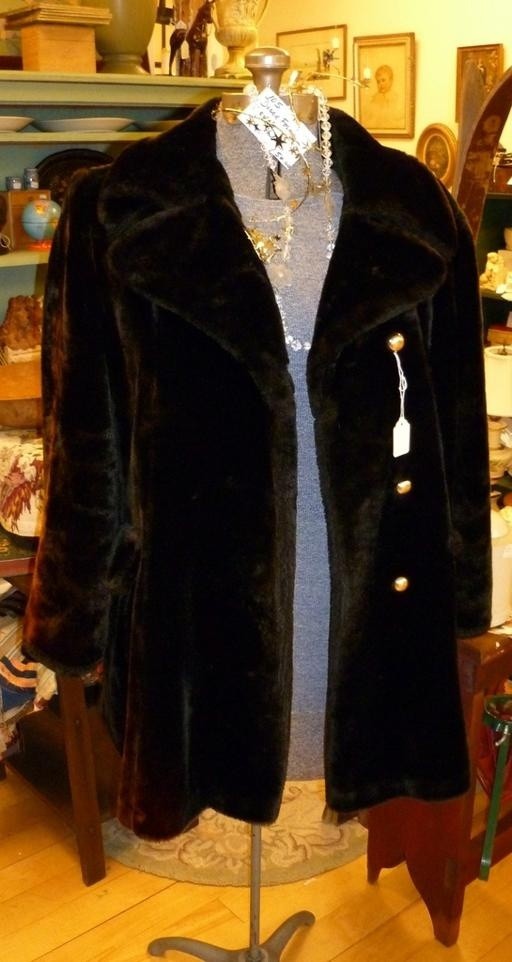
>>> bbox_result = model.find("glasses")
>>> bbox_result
[483,342,512,480]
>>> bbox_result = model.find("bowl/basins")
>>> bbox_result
[260,74,342,355]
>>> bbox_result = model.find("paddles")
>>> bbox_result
[99,778,364,887]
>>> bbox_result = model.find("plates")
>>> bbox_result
[0,67,271,883]
[476,192,512,303]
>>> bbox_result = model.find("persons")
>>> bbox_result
[36,42,498,812]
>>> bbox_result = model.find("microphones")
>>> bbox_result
[354,628,512,947]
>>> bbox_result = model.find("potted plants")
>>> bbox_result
[350,31,416,138]
[274,22,348,100]
[455,41,506,124]
[416,121,459,189]
